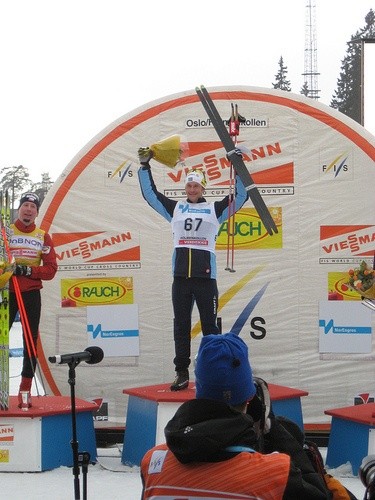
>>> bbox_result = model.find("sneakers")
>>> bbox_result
[171,374,189,391]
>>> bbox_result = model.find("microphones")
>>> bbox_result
[49,345,105,366]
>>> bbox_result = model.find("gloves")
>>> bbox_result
[14,265,27,275]
[138,147,152,165]
[226,149,243,162]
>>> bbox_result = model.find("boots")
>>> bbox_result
[18,376,32,412]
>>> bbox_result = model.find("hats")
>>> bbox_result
[185,170,207,190]
[194,333,257,404]
[20,192,40,207]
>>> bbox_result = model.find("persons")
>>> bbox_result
[8,192,58,411]
[138,147,248,391]
[142,333,357,500]
